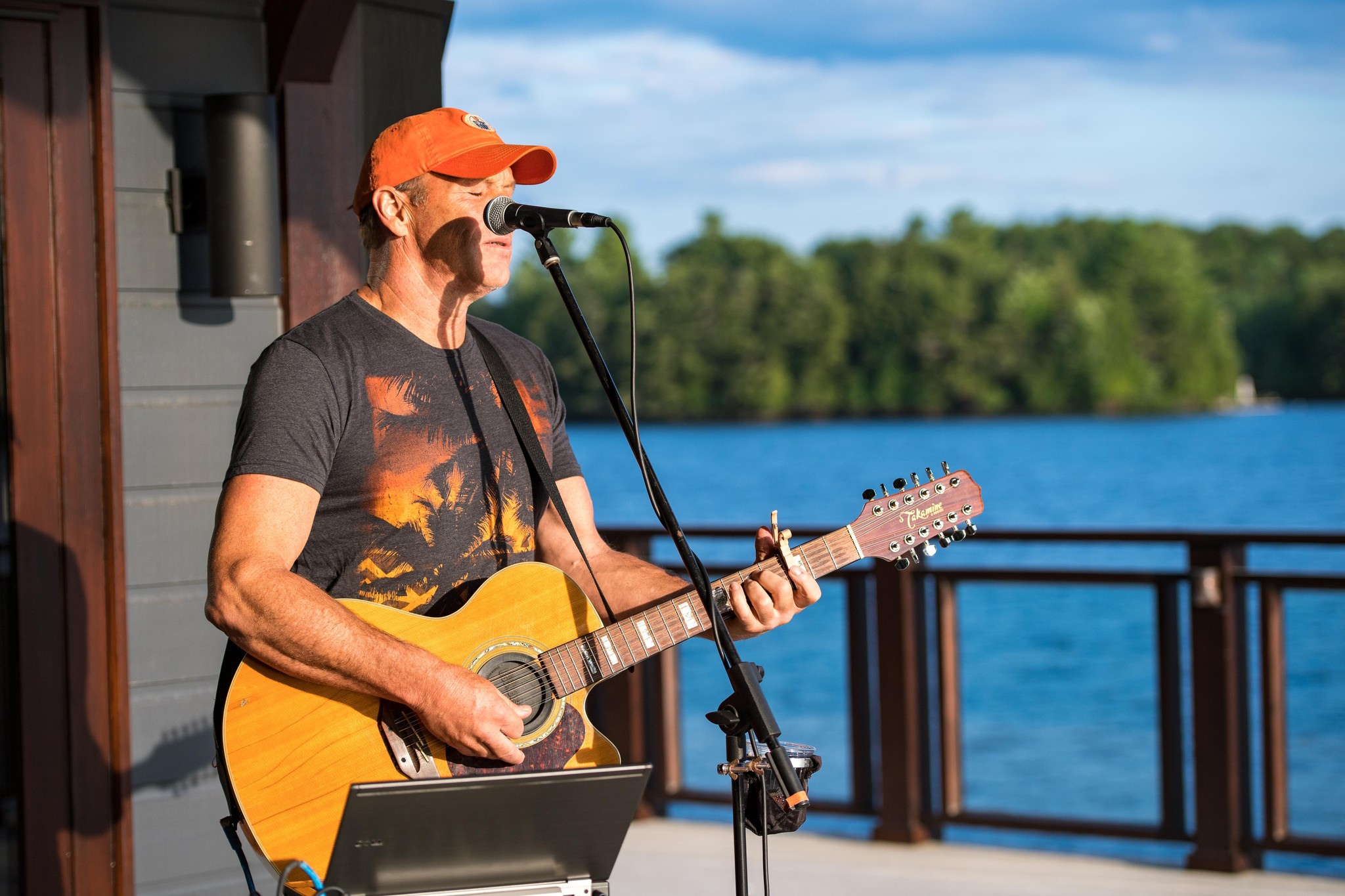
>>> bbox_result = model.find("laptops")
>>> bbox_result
[284,763,655,896]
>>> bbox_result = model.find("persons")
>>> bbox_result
[205,107,821,896]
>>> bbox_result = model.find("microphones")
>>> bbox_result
[483,195,612,236]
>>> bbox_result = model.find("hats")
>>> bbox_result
[369,107,556,185]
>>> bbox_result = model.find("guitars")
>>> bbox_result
[213,461,984,896]
